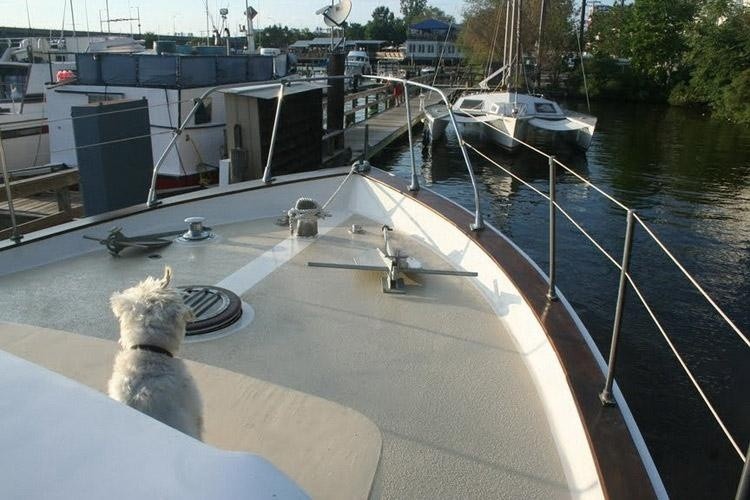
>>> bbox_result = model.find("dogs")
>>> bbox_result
[108,266,203,442]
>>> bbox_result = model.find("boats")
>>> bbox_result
[201,0,407,96]
[1,1,149,182]
[0,0,351,245]
[0,69,750,500]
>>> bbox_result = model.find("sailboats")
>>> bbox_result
[422,0,599,159]
[418,145,593,238]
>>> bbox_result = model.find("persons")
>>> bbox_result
[392,80,402,107]
